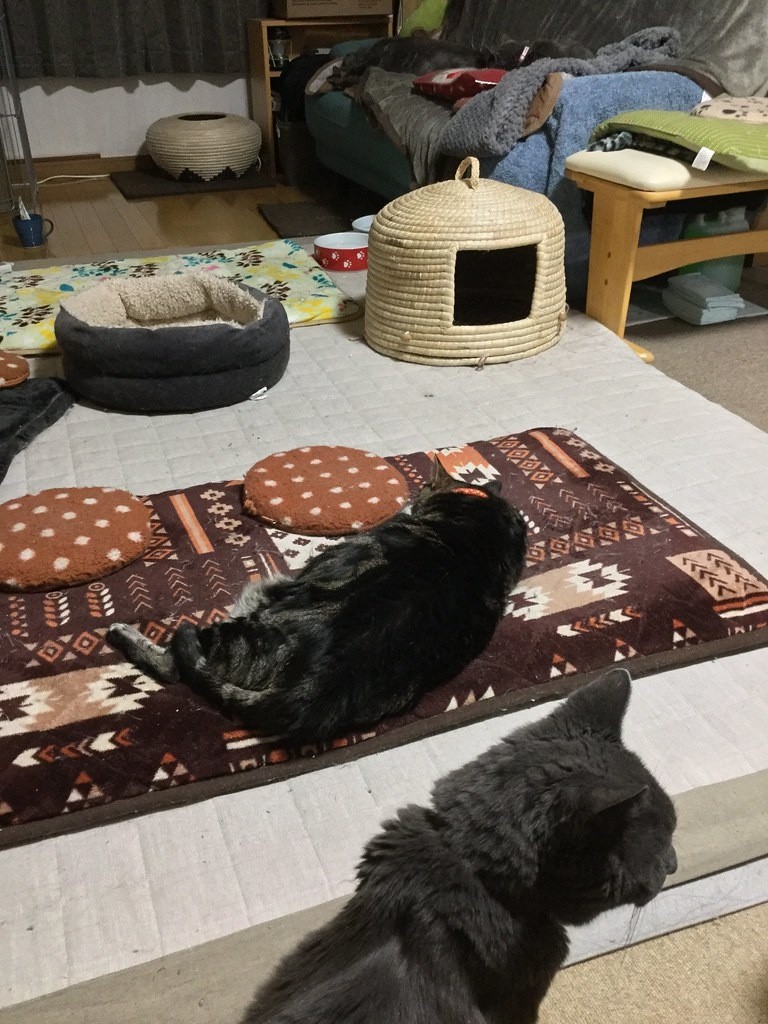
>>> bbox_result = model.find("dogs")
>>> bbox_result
[102,451,529,753]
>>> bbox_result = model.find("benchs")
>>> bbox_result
[558,145,768,362]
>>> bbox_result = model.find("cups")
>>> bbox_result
[12,214,54,249]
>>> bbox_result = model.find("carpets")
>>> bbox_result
[0,236,768,1024]
[255,196,381,239]
[0,235,364,355]
[109,169,277,201]
[0,427,768,852]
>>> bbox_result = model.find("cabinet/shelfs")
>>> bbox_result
[246,14,395,180]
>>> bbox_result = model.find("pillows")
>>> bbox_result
[411,66,508,101]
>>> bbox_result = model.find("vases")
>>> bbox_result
[147,113,263,183]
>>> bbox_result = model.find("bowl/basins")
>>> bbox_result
[313,232,372,271]
[352,214,378,233]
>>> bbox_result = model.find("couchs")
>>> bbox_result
[304,0,768,303]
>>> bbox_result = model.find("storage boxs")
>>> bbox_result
[271,0,393,20]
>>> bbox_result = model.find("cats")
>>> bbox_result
[240,666,679,1021]
[484,32,597,72]
[365,28,499,76]
